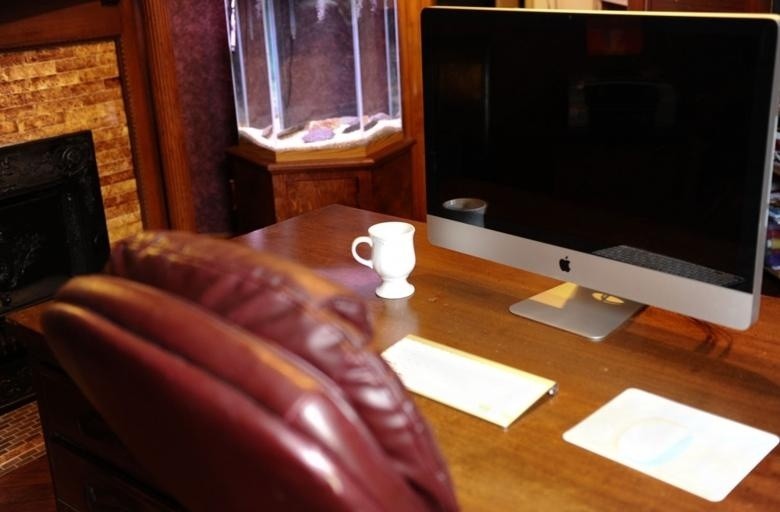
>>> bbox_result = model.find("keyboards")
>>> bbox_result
[378,333,559,430]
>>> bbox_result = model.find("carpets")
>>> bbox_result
[0,454,57,512]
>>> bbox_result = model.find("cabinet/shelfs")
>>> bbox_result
[231,143,416,230]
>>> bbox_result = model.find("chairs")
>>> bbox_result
[28,228,459,512]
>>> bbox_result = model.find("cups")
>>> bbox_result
[351,221,416,299]
[441,196,490,230]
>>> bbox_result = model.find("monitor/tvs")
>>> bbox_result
[420,5,780,340]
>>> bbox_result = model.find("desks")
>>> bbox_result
[222,203,779,512]
